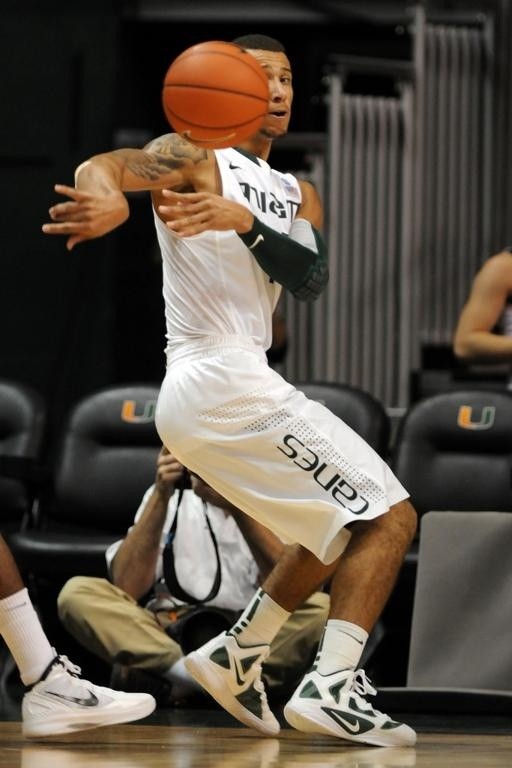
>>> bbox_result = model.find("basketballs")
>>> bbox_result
[163,41,271,149]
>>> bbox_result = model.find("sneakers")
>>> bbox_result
[14,651,161,740]
[278,659,418,749]
[177,628,286,740]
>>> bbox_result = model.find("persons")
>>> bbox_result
[54,438,384,716]
[451,234,512,392]
[0,533,159,741]
[40,33,421,752]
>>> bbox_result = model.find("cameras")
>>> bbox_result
[174,467,198,489]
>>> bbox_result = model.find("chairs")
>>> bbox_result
[0,383,512,727]
